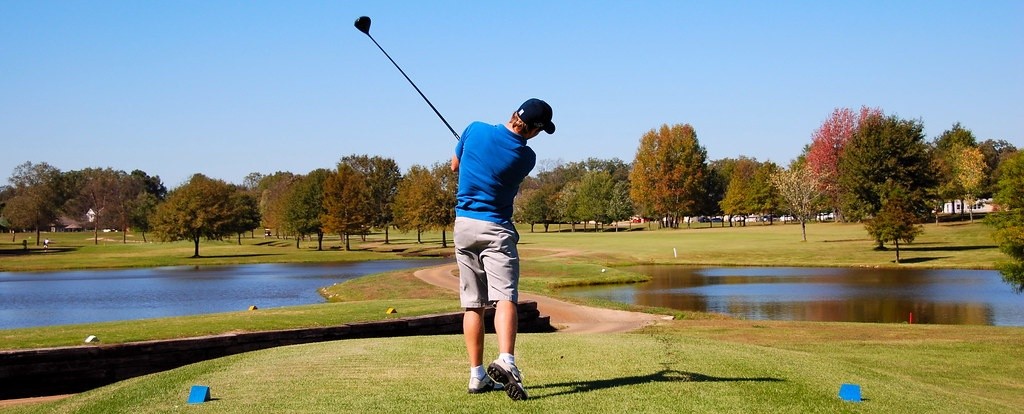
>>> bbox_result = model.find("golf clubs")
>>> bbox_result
[354,15,463,142]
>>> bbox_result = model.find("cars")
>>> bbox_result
[698,217,722,223]
[725,212,835,222]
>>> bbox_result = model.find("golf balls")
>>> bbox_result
[601,268,606,273]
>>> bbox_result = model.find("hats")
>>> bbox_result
[516,98,556,135]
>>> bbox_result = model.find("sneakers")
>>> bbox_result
[486,359,528,402]
[468,372,505,394]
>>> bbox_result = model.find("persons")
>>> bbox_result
[451,98,556,401]
[43,238,49,252]
